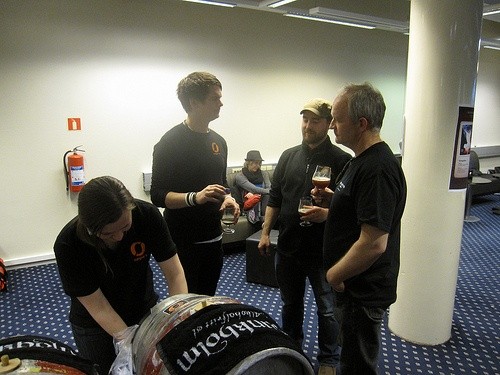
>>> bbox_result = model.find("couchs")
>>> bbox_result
[220,169,277,245]
[467,151,500,195]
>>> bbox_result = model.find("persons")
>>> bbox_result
[258,99,351,375]
[54,176,188,375]
[311,83,407,375]
[235,151,271,230]
[151,73,240,296]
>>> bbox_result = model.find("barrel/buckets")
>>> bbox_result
[130,293,317,375]
[0,334,89,375]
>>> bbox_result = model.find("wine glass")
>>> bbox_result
[312,164,331,190]
[222,207,236,235]
[298,197,314,227]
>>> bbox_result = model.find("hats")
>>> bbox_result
[244,150,265,161]
[300,100,332,118]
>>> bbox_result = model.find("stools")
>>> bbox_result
[246,228,279,287]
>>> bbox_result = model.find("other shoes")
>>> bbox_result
[317,364,337,375]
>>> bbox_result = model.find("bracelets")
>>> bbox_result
[185,192,196,207]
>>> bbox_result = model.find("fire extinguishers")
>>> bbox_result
[63,145,85,192]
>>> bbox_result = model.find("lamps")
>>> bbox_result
[184,0,409,33]
[481,2,500,51]
